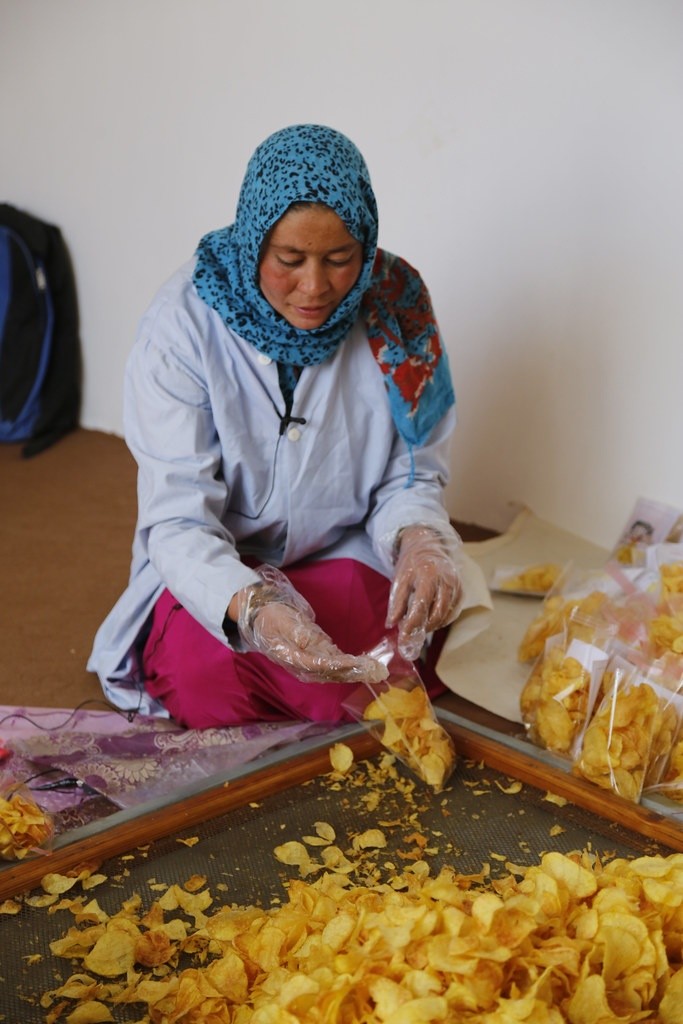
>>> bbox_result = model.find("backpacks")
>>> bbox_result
[0,204,82,458]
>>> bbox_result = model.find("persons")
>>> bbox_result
[86,123,465,730]
